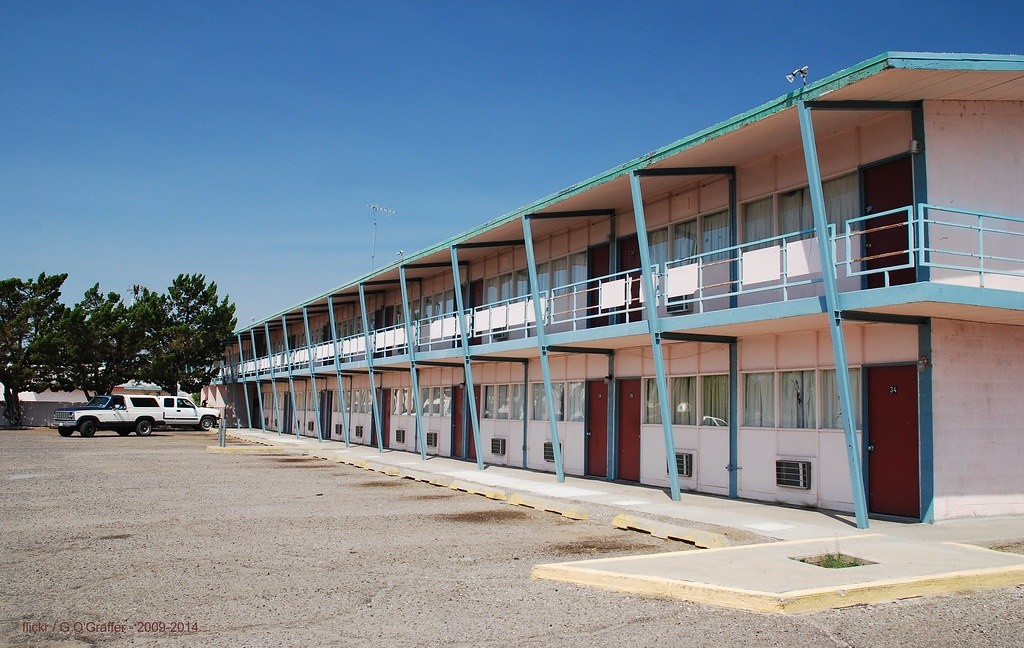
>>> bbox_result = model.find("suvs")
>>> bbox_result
[52,393,167,438]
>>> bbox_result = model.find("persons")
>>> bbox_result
[202,397,208,407]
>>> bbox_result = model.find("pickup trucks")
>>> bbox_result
[100,394,221,431]
